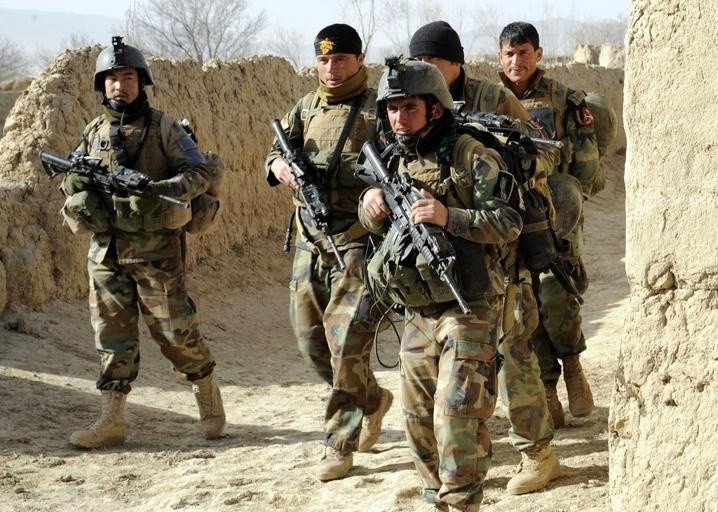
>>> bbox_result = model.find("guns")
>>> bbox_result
[271,119,347,271]
[551,255,584,306]
[353,140,473,316]
[38,150,191,209]
[451,99,565,160]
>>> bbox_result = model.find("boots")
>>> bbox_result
[187,369,225,440]
[68,387,127,448]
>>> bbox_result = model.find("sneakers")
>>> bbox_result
[505,443,559,496]
[318,444,352,480]
[563,365,595,418]
[543,388,568,427]
[355,388,393,451]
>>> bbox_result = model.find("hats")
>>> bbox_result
[313,24,361,59]
[409,20,464,68]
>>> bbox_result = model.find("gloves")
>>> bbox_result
[64,173,95,196]
[128,181,169,213]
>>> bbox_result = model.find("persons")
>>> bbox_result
[495,23,599,427]
[358,55,522,511]
[409,21,562,494]
[264,24,393,481]
[58,35,225,448]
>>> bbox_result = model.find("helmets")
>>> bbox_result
[93,44,155,93]
[372,62,456,139]
